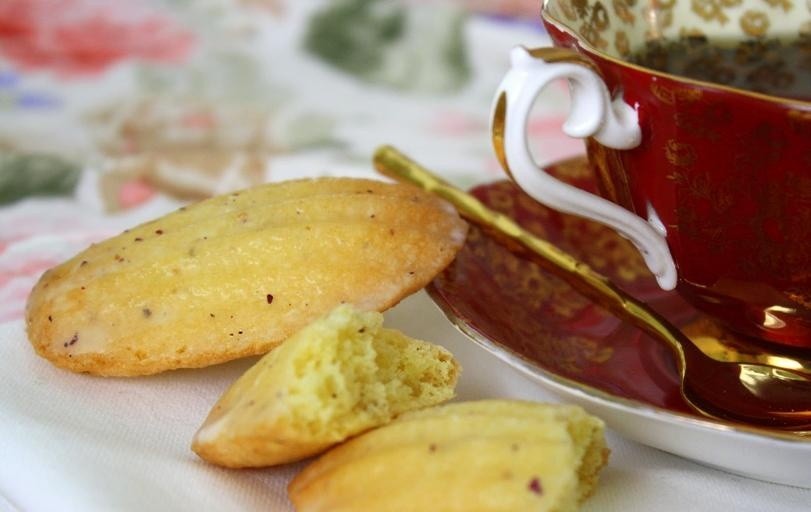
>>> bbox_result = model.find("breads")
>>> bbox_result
[21,167,615,512]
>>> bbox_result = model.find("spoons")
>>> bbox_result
[363,139,810,441]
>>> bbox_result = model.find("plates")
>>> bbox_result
[423,154,811,499]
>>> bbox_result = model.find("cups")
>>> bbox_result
[491,3,808,361]
